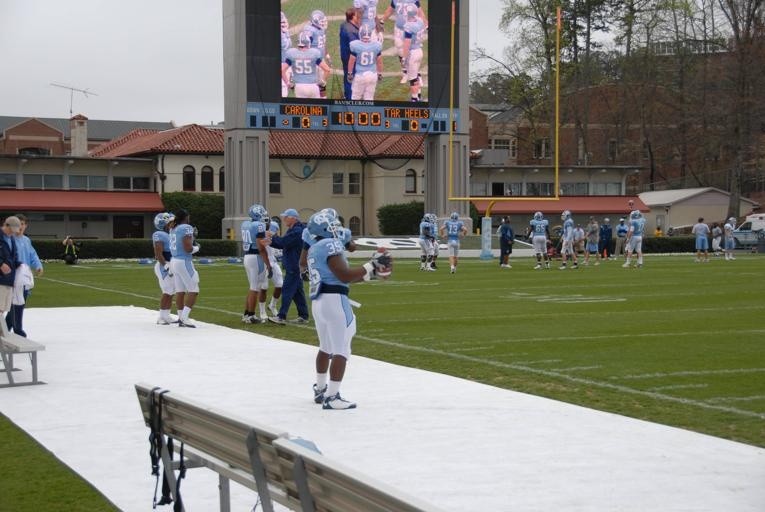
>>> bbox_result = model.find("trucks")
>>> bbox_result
[733,213,765,249]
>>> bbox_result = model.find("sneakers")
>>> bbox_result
[267,303,278,316]
[169,315,180,323]
[268,315,286,325]
[421,261,649,274]
[245,315,260,323]
[322,391,358,409]
[313,382,328,404]
[288,317,309,324]
[399,75,409,84]
[241,313,248,322]
[178,320,198,329]
[260,311,268,320]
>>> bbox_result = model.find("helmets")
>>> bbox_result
[359,25,373,43]
[422,213,438,222]
[296,31,314,48]
[247,204,268,222]
[167,212,177,222]
[310,9,329,32]
[630,210,640,219]
[280,12,288,32]
[533,211,544,221]
[561,211,571,220]
[451,212,459,221]
[320,208,341,220]
[403,4,419,18]
[153,211,171,231]
[308,213,343,240]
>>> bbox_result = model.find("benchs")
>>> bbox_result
[130,376,285,512]
[0,284,49,391]
[269,434,453,512]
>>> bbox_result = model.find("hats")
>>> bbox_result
[4,216,22,233]
[278,208,301,218]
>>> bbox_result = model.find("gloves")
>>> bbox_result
[325,54,332,66]
[399,57,406,67]
[379,19,385,28]
[346,72,353,83]
[286,81,295,90]
[298,268,311,282]
[266,267,274,280]
[363,251,392,275]
[194,240,203,255]
[364,270,382,281]
[164,262,173,277]
[376,72,384,83]
[316,80,327,92]
[377,33,384,44]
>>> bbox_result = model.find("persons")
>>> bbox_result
[169,210,201,329]
[499,215,515,269]
[0,216,20,315]
[723,214,737,261]
[240,204,356,326]
[525,210,647,271]
[497,218,505,242]
[712,222,724,257]
[153,212,176,325]
[655,226,664,236]
[5,214,43,337]
[692,217,711,263]
[61,234,79,264]
[305,212,391,411]
[281,0,429,103]
[440,212,467,273]
[418,213,436,272]
[427,214,438,268]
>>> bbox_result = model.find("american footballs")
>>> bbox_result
[377,247,391,279]
